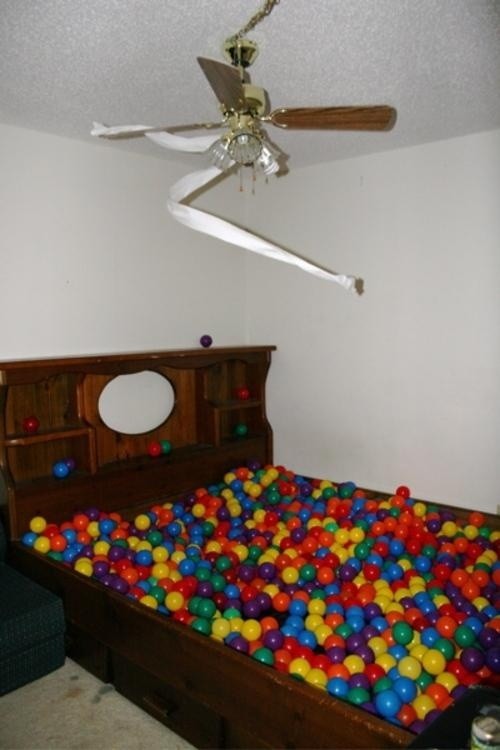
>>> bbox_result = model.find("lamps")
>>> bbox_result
[207,115,292,194]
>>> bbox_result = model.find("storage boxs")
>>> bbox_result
[2,559,67,696]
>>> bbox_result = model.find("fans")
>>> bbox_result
[98,39,398,141]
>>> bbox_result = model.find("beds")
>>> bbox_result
[1,346,500,750]
